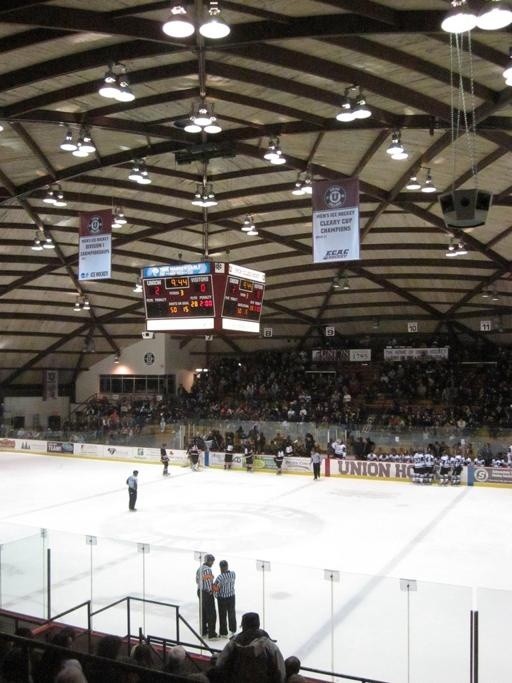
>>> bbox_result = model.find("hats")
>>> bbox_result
[239,613,260,629]
[203,554,228,567]
[168,646,219,663]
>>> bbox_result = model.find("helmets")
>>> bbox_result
[367,447,482,460]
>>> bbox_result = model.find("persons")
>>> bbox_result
[126,471,138,512]
[284,656,308,683]
[212,560,236,638]
[196,554,220,638]
[2,338,512,487]
[0,613,286,683]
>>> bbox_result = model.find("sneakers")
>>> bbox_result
[202,627,236,638]
[411,480,460,486]
